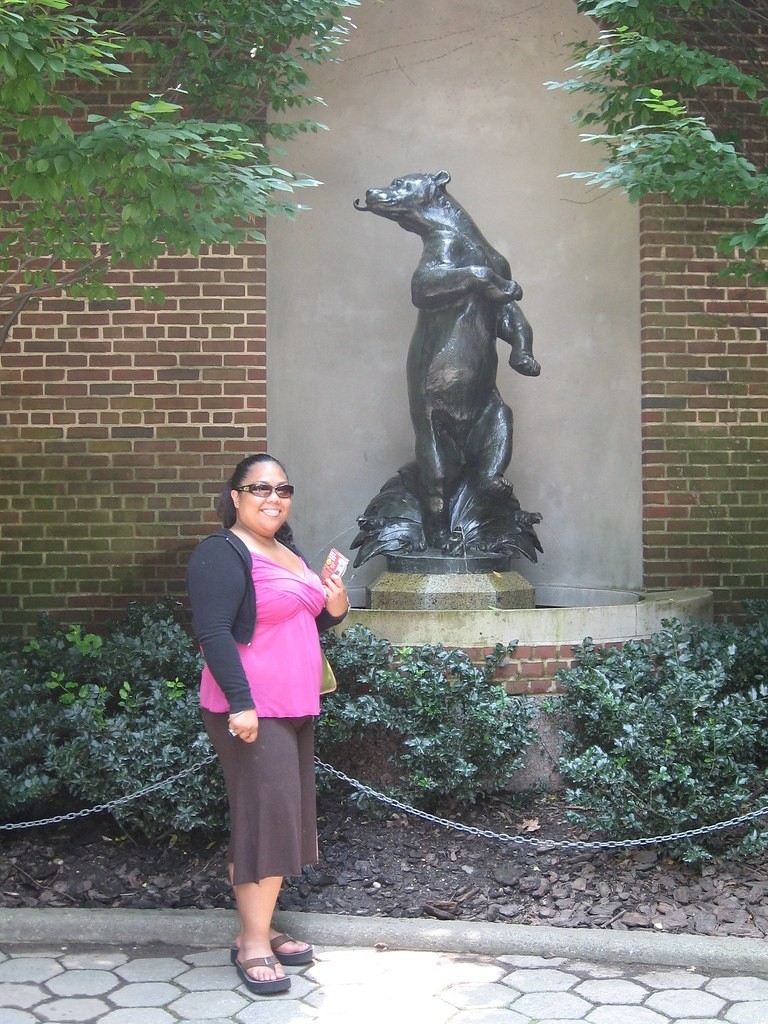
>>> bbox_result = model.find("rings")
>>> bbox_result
[228,730,237,737]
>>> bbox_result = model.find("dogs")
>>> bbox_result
[353,168,545,549]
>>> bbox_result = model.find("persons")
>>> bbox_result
[185,453,351,994]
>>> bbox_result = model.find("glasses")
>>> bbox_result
[236,483,294,499]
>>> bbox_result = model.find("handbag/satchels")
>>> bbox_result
[318,647,337,696]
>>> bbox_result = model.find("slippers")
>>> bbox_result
[230,932,313,965]
[234,956,292,995]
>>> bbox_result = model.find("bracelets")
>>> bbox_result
[229,711,243,718]
